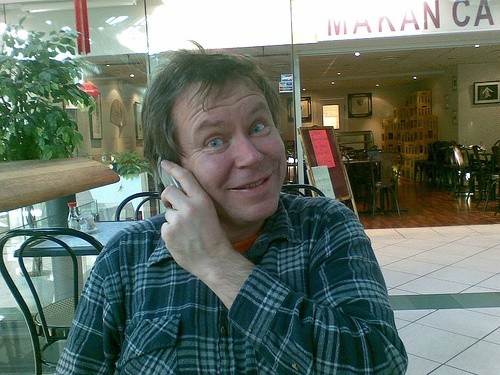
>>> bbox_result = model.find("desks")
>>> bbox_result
[344,158,409,213]
[13,220,141,257]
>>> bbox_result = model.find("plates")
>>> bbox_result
[342,159,353,161]
[79,228,98,233]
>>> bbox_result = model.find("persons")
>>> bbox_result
[55,39,408,375]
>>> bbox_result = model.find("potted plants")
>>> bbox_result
[89,149,154,204]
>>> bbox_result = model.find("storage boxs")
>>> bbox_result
[380,90,437,182]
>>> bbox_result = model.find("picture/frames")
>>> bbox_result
[473,80,500,104]
[135,102,142,140]
[89,93,103,140]
[348,93,373,118]
[287,97,312,122]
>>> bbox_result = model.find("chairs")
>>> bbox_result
[414,140,500,216]
[0,191,162,375]
[341,146,402,219]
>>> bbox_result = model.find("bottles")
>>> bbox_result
[66,202,80,230]
[79,213,94,230]
